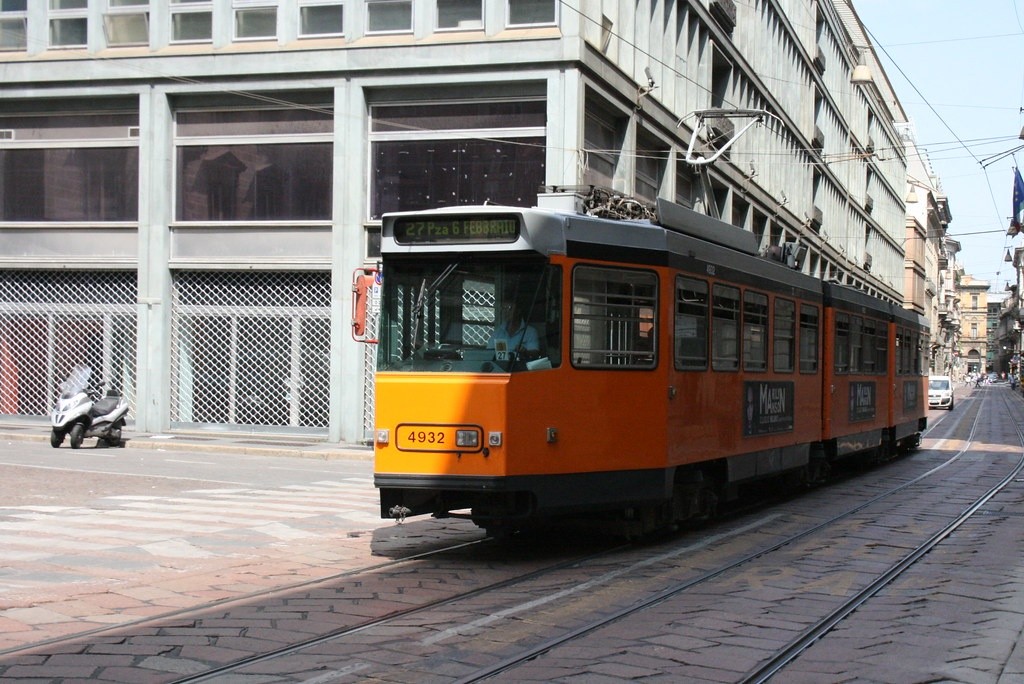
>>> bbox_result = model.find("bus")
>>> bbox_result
[348,104,931,543]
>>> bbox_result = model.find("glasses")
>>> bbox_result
[503,304,517,310]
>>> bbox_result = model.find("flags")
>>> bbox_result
[1012,171,1024,223]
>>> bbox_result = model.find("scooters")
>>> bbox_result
[47,365,132,450]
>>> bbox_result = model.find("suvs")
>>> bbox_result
[929,375,954,411]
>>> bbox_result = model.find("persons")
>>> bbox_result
[1001,371,1006,380]
[965,375,971,387]
[959,373,962,383]
[975,374,980,387]
[486,296,539,360]
[1009,375,1016,390]
